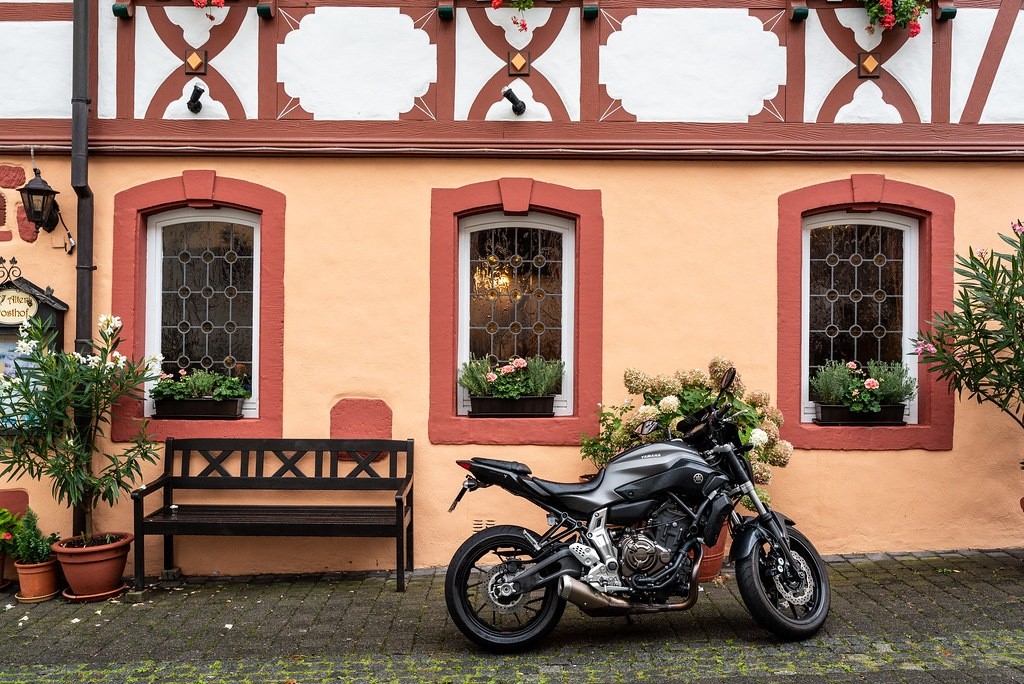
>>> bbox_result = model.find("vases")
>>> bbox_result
[468,393,555,417]
[813,402,908,424]
[153,394,246,419]
[53,531,134,597]
[694,518,729,577]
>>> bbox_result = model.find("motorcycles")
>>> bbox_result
[444,367,832,656]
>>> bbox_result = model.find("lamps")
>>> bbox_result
[12,168,62,235]
[584,6,598,23]
[500,86,526,114]
[257,4,274,18]
[937,6,956,24]
[112,5,134,19]
[788,6,808,22]
[439,5,455,23]
[186,84,207,114]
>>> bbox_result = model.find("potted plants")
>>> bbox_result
[13,504,62,603]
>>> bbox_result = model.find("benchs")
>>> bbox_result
[128,434,417,593]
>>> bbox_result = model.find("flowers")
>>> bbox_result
[817,359,918,411]
[491,0,533,32]
[0,315,163,544]
[0,503,19,562]
[865,0,928,38]
[149,367,246,401]
[576,354,792,514]
[193,0,224,19]
[455,351,567,397]
[905,217,1024,474]
